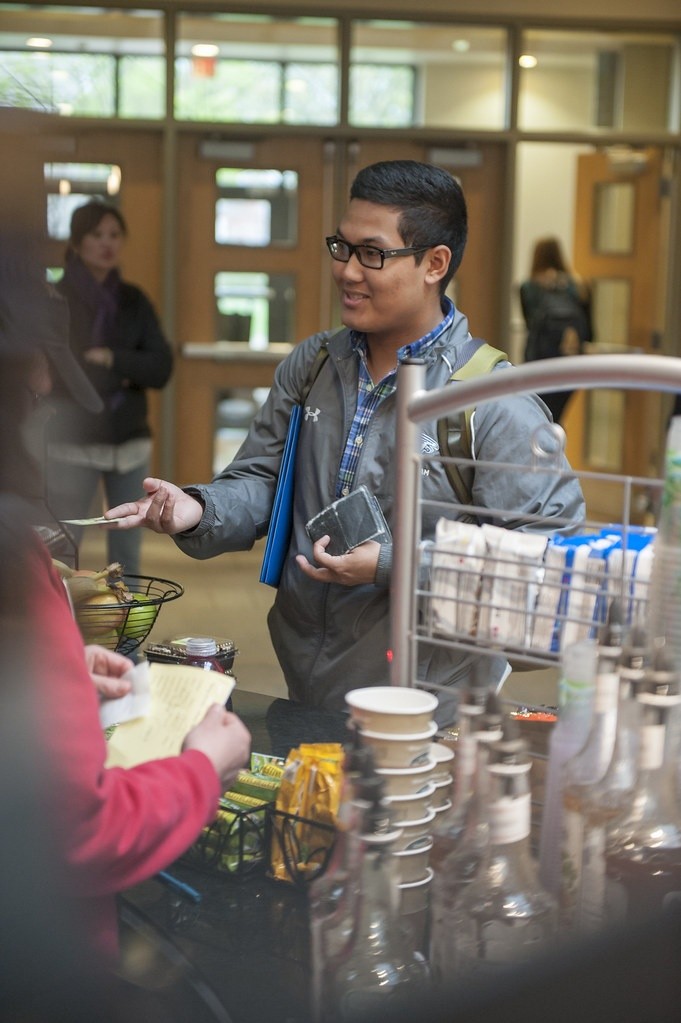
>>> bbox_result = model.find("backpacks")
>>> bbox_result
[529,289,589,360]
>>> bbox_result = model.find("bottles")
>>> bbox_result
[292,599,681,1023]
[178,638,234,715]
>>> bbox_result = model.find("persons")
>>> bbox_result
[0,256,254,981]
[41,203,173,661]
[520,238,596,426]
[98,159,586,741]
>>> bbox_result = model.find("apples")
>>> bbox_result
[74,571,156,651]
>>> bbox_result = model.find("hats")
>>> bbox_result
[0,247,106,414]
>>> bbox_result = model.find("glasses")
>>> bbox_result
[24,384,51,410]
[325,234,430,270]
